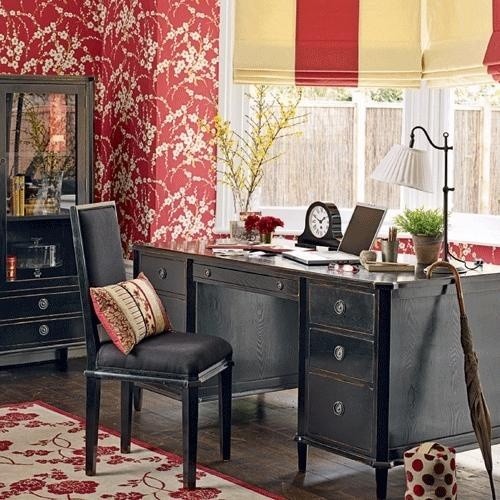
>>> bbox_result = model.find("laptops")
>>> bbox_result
[282,201,388,267]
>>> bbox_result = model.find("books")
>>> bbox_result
[6,256,16,281]
[10,176,25,216]
[360,261,415,272]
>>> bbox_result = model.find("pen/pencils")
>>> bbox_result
[389,226,398,242]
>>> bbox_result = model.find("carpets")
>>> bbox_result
[0,399,288,499]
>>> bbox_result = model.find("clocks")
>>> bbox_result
[295,201,343,252]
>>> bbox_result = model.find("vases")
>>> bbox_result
[230,221,246,238]
[39,178,62,214]
[260,232,272,245]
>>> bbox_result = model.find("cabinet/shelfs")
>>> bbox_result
[0,73,99,371]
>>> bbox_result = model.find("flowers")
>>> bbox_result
[243,215,284,234]
[15,92,75,202]
[196,83,310,221]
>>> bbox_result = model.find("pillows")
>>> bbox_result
[89,272,177,357]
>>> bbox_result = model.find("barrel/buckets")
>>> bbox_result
[412,233,442,262]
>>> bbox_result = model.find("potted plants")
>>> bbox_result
[391,205,452,264]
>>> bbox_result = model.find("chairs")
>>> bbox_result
[70,200,234,490]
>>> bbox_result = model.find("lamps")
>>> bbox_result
[371,125,465,274]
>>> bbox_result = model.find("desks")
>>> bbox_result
[127,240,500,500]
